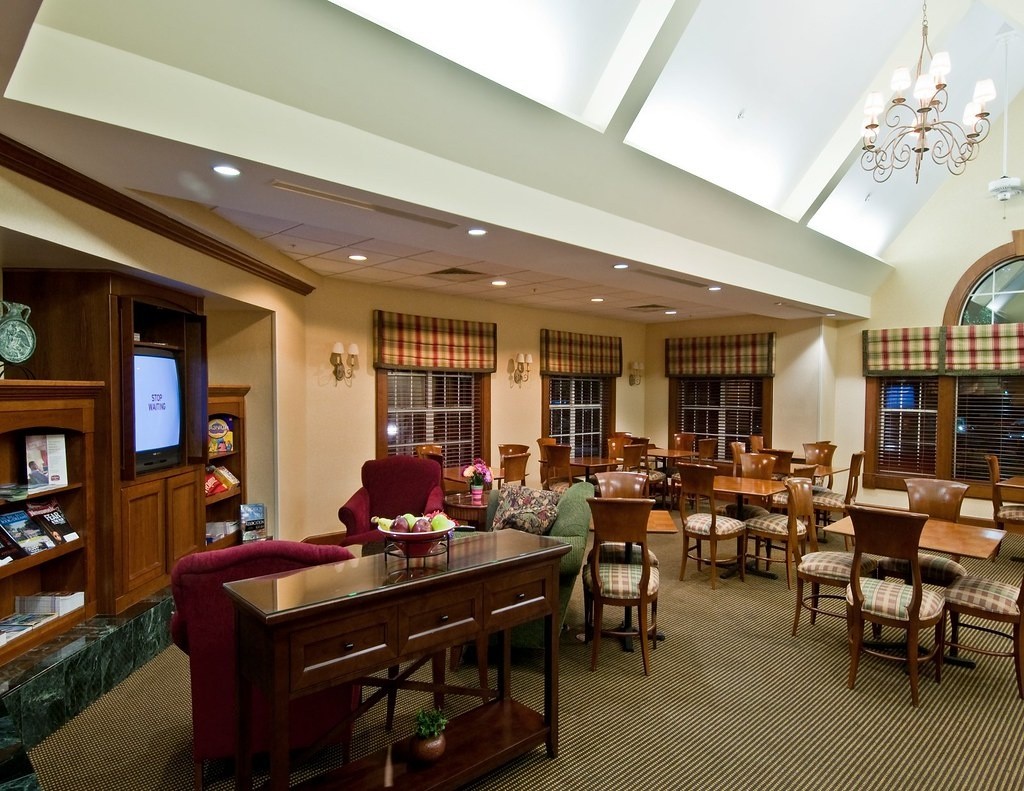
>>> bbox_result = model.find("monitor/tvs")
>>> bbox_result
[133,346,186,472]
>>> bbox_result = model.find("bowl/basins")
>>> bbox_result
[377,517,455,557]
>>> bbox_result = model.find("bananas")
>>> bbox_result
[370,516,393,530]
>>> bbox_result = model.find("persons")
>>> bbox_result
[29,461,49,484]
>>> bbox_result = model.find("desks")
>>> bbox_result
[995,474,1024,562]
[674,474,788,581]
[539,456,623,483]
[443,466,505,490]
[222,527,574,791]
[821,513,1007,671]
[642,449,699,511]
[789,463,850,545]
[588,510,679,654]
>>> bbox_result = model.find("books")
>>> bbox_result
[0,590,84,647]
[0,433,69,501]
[207,416,234,452]
[206,503,268,545]
[205,464,240,496]
[0,499,79,565]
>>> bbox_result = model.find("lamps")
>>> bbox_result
[513,352,533,385]
[331,340,358,381]
[629,360,645,386]
[860,0,996,185]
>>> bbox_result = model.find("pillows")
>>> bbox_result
[485,482,562,535]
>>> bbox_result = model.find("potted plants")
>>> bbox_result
[412,705,450,762]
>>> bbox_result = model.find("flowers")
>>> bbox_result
[461,457,494,485]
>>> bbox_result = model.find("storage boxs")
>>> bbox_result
[212,466,239,491]
[208,414,234,452]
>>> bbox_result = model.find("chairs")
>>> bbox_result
[168,431,1024,791]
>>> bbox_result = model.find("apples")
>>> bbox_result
[389,513,450,533]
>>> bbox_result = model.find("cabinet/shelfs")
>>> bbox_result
[0,267,212,615]
[0,379,107,668]
[207,383,252,549]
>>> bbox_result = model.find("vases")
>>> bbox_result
[471,485,484,505]
